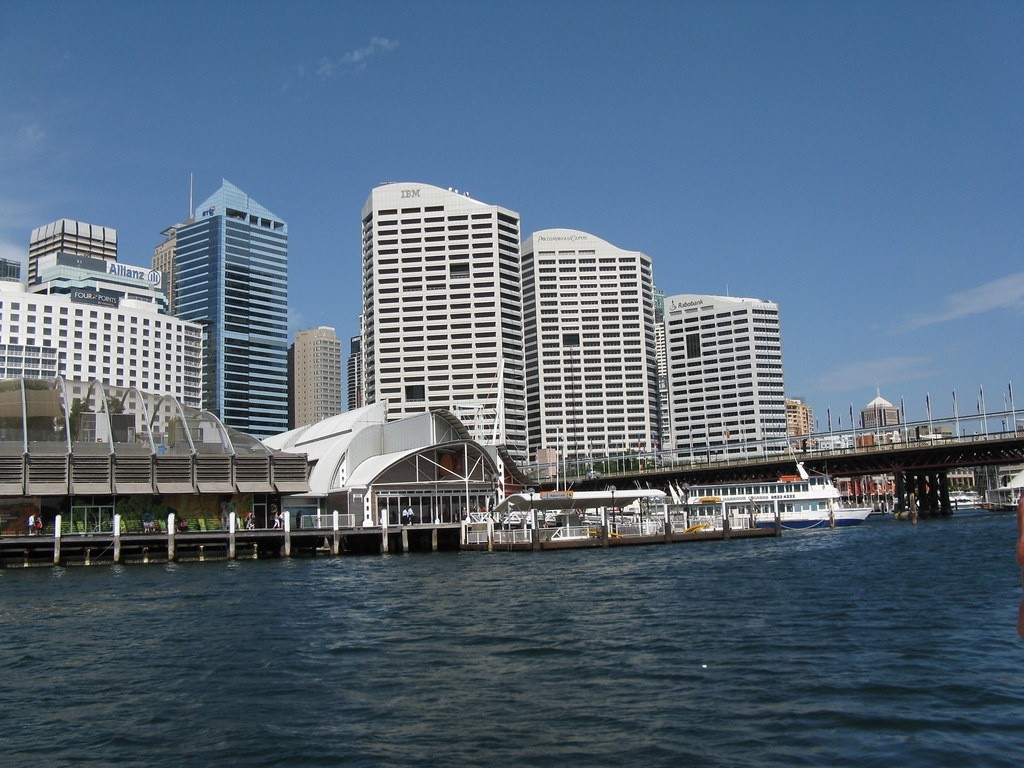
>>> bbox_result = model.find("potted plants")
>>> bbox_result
[101,395,126,413]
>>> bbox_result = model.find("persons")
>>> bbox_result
[246,510,255,531]
[456,503,486,522]
[296,510,302,529]
[402,506,415,526]
[174,519,189,533]
[143,519,156,534]
[28,511,43,536]
[273,509,286,531]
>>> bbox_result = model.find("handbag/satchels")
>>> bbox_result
[37,523,41,528]
[251,514,255,524]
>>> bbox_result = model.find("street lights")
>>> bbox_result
[608,484,617,533]
[527,487,534,510]
[484,472,498,519]
[682,483,689,530]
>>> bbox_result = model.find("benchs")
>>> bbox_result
[62,514,253,534]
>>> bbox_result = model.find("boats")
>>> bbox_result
[684,435,876,530]
